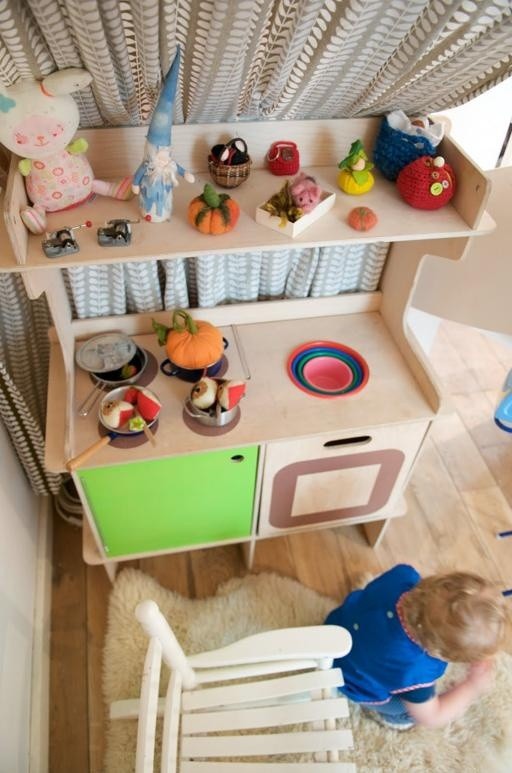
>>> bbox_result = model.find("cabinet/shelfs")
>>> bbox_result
[0,116,498,585]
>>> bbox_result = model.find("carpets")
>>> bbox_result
[98,568,510,772]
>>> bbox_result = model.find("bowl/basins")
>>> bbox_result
[55,478,82,526]
[285,340,370,399]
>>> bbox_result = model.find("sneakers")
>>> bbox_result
[360,696,420,732]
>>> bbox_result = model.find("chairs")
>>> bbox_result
[106,602,359,772]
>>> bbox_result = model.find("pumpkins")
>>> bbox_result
[168,307,224,370]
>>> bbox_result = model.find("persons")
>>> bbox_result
[320,563,508,739]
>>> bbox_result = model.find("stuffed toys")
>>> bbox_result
[267,141,299,176]
[42,109,459,259]
[347,207,377,232]
[374,109,458,210]
[0,67,141,237]
[131,43,195,224]
[290,171,321,210]
[209,141,248,166]
[337,139,375,195]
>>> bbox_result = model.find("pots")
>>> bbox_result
[65,334,238,472]
